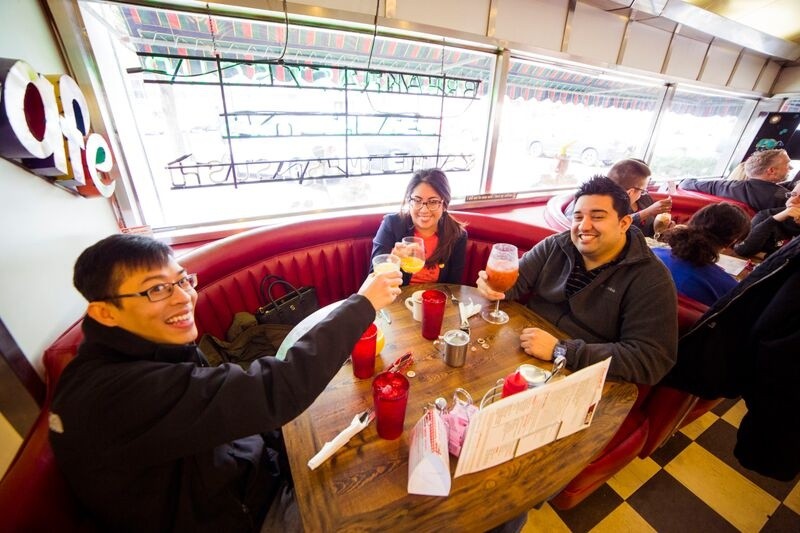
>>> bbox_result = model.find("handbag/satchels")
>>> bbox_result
[256,274,320,327]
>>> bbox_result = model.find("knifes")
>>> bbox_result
[381,306,393,324]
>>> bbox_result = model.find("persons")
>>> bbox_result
[368,167,467,286]
[48,233,404,533]
[734,179,800,256]
[652,202,752,308]
[565,149,790,242]
[476,174,679,414]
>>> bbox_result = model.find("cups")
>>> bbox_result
[434,329,471,368]
[350,323,378,379]
[401,237,426,272]
[372,369,410,440]
[405,289,430,321]
[371,253,401,290]
[421,290,436,349]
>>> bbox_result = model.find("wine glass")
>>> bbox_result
[481,242,520,325]
[647,213,671,244]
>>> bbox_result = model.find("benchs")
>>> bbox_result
[0,182,790,533]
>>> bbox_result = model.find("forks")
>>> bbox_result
[445,284,460,304]
[358,406,371,423]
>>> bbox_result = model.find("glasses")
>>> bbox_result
[631,186,648,196]
[95,273,198,303]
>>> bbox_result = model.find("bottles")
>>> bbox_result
[502,371,528,399]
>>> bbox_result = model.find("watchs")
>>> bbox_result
[551,338,569,363]
